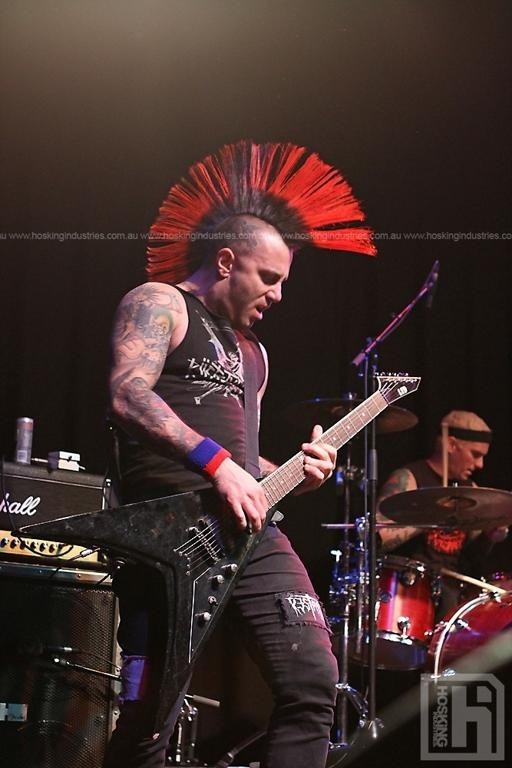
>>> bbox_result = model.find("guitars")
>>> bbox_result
[10,369,422,679]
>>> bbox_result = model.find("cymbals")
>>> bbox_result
[379,486,512,531]
[278,399,420,435]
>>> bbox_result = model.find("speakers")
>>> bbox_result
[0,562,115,768]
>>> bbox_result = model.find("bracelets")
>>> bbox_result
[184,437,228,476]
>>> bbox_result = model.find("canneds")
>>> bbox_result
[13,417,34,465]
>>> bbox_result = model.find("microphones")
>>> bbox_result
[32,645,83,655]
[425,263,439,310]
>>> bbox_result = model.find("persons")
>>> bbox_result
[105,205,338,768]
[370,408,510,619]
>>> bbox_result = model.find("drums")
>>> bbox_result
[331,554,442,671]
[426,571,512,686]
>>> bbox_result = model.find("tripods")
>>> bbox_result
[215,437,388,768]
